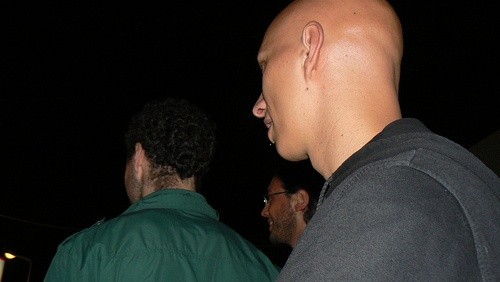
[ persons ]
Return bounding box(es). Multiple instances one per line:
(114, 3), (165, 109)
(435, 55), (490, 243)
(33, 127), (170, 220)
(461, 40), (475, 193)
(259, 159), (327, 252)
(250, 0), (500, 282)
(41, 94), (281, 282)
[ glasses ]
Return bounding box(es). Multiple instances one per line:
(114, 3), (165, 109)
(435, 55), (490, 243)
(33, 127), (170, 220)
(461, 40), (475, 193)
(264, 190), (292, 205)
